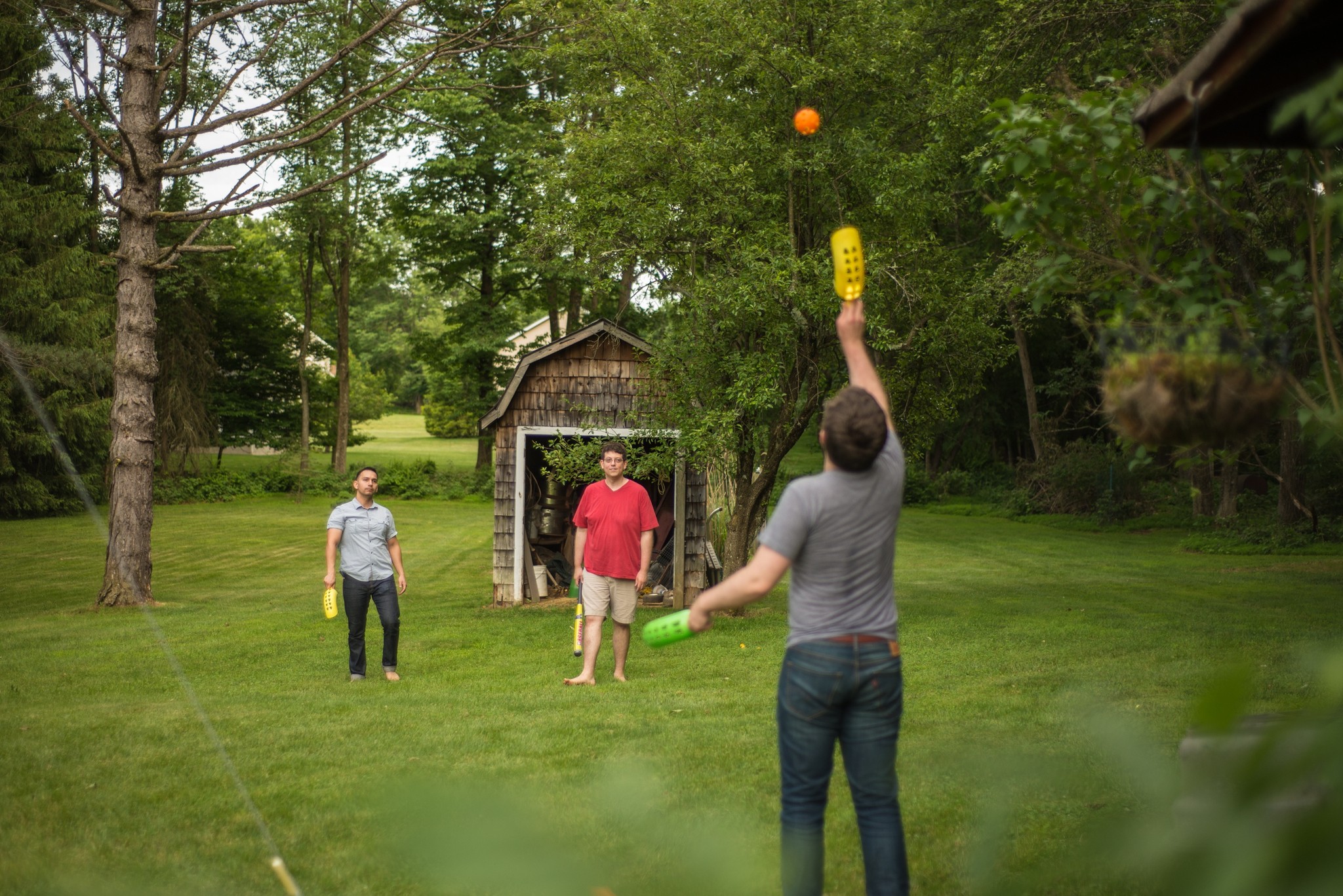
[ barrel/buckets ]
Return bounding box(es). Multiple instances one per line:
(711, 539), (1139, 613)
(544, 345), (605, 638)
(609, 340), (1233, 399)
(567, 574), (579, 598)
(524, 565), (548, 598)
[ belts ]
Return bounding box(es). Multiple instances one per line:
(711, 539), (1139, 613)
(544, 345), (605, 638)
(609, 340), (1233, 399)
(825, 634), (884, 643)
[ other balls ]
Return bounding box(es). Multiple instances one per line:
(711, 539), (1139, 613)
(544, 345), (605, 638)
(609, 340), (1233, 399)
(794, 109), (820, 135)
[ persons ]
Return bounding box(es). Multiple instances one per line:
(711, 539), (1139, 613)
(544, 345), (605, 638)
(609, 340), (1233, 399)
(323, 467), (407, 684)
(562, 449), (660, 686)
(685, 296), (914, 895)
